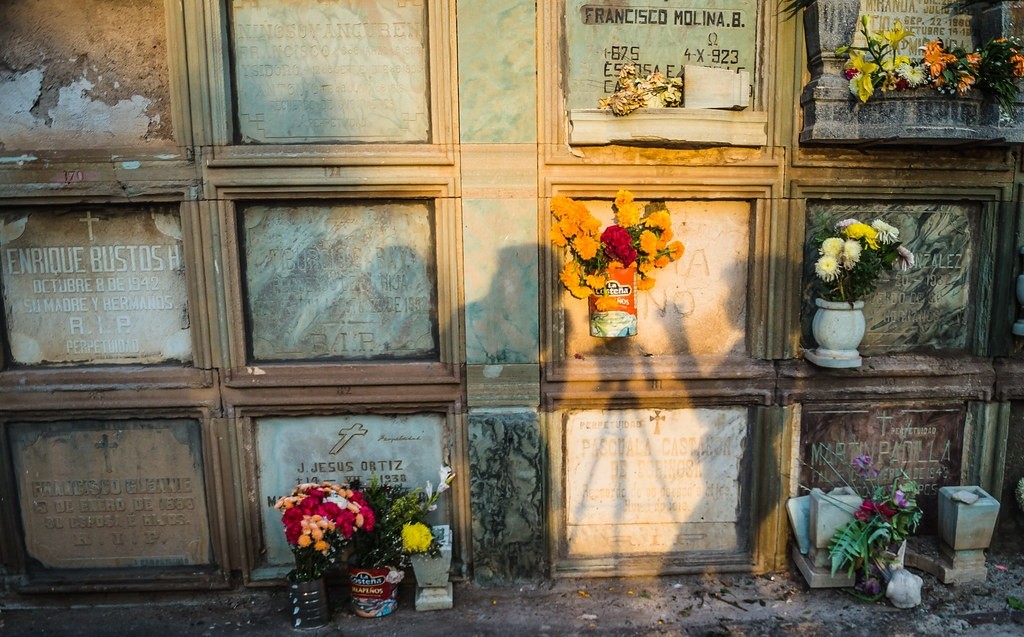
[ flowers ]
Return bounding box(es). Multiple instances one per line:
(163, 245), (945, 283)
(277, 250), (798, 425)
(549, 186), (686, 312)
(598, 61), (683, 117)
(832, 9), (1024, 117)
(792, 436), (923, 603)
(806, 216), (914, 309)
(344, 464), (457, 586)
(273, 479), (377, 583)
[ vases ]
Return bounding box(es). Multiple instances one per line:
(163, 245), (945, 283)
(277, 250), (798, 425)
(586, 267), (638, 338)
(343, 566), (399, 619)
(681, 63), (750, 109)
(886, 543), (925, 609)
(286, 568), (331, 631)
(811, 297), (866, 360)
